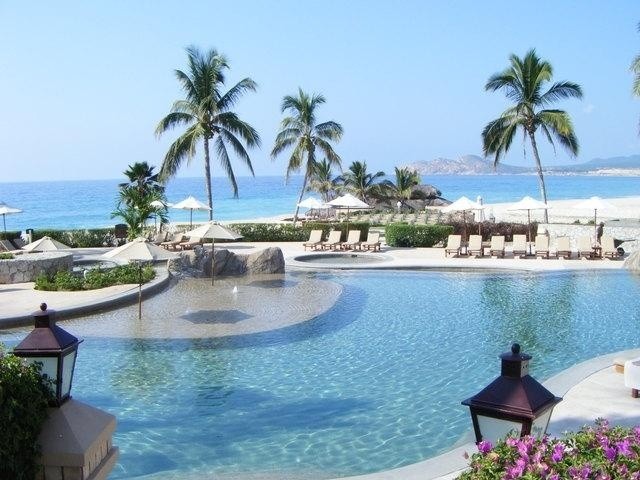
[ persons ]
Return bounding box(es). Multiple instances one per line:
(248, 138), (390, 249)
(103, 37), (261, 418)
(597, 222), (605, 245)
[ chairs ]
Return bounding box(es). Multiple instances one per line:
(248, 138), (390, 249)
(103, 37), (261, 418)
(302, 229), (381, 252)
(444, 234), (619, 261)
(151, 233), (203, 251)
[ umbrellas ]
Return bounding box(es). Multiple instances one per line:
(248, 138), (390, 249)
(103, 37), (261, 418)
(0, 204), (23, 231)
(574, 196), (617, 255)
(22, 236), (71, 253)
(506, 196), (551, 255)
(171, 195), (212, 232)
(443, 196), (485, 255)
(296, 192), (371, 241)
(180, 220), (245, 286)
(101, 236), (182, 319)
(134, 199), (174, 231)
